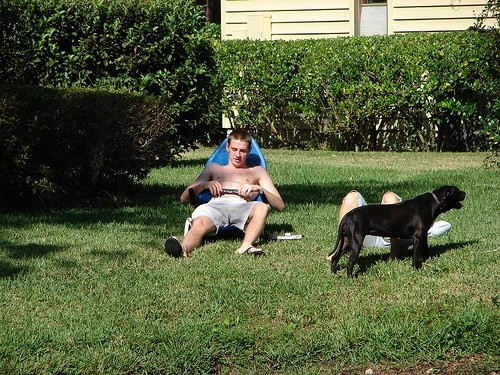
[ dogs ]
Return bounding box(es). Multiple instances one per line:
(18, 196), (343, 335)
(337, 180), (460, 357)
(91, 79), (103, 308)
(327, 185), (466, 280)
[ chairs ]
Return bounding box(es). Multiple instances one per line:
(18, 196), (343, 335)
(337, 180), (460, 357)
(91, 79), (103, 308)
(197, 133), (267, 230)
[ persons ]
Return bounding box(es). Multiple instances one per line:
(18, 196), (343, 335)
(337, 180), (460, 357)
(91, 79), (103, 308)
(163, 127), (284, 258)
(337, 189), (453, 249)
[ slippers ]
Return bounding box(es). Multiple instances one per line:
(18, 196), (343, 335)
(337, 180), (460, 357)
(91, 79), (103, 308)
(165, 236), (187, 260)
(234, 246), (264, 255)
